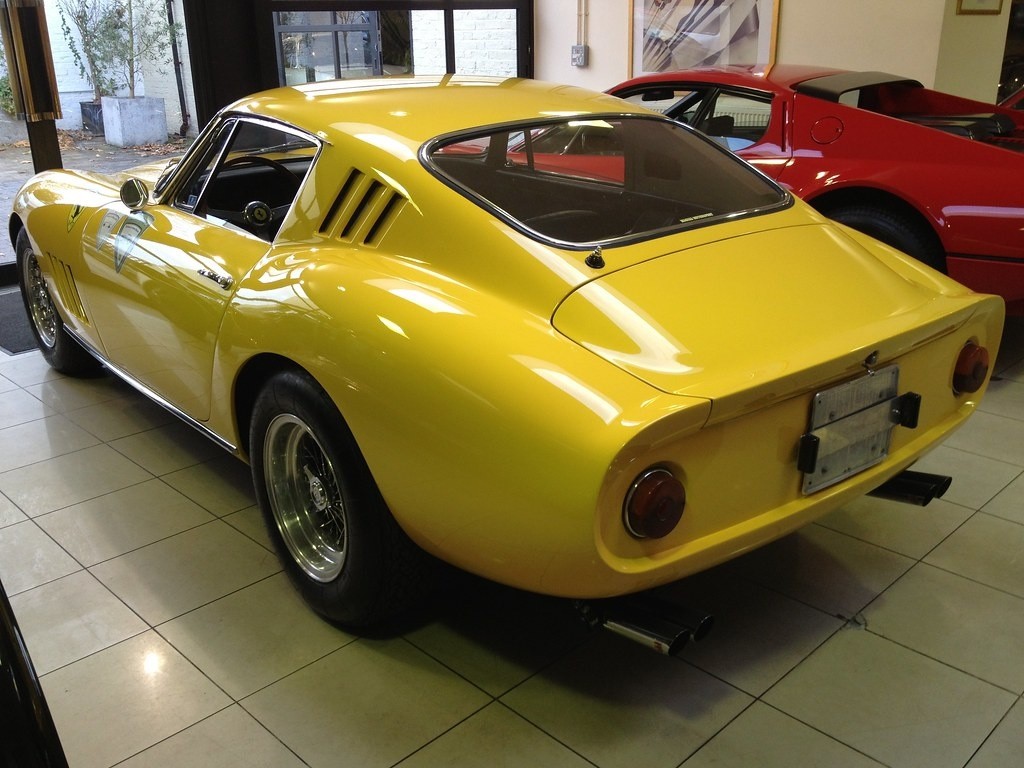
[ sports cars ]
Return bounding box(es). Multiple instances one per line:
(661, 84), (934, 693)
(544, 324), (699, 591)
(7, 70), (1006, 656)
(433, 63), (1024, 311)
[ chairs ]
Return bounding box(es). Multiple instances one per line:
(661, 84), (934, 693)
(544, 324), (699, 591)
(521, 208), (607, 245)
(700, 115), (736, 139)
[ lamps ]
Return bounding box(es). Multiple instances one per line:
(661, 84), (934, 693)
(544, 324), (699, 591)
(570, 45), (588, 68)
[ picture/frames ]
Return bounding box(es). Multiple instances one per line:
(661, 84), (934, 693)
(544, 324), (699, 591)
(956, 0), (1003, 16)
(627, 0), (781, 82)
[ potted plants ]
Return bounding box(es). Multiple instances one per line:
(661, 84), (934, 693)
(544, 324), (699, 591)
(60, 0), (136, 137)
(100, 0), (181, 149)
